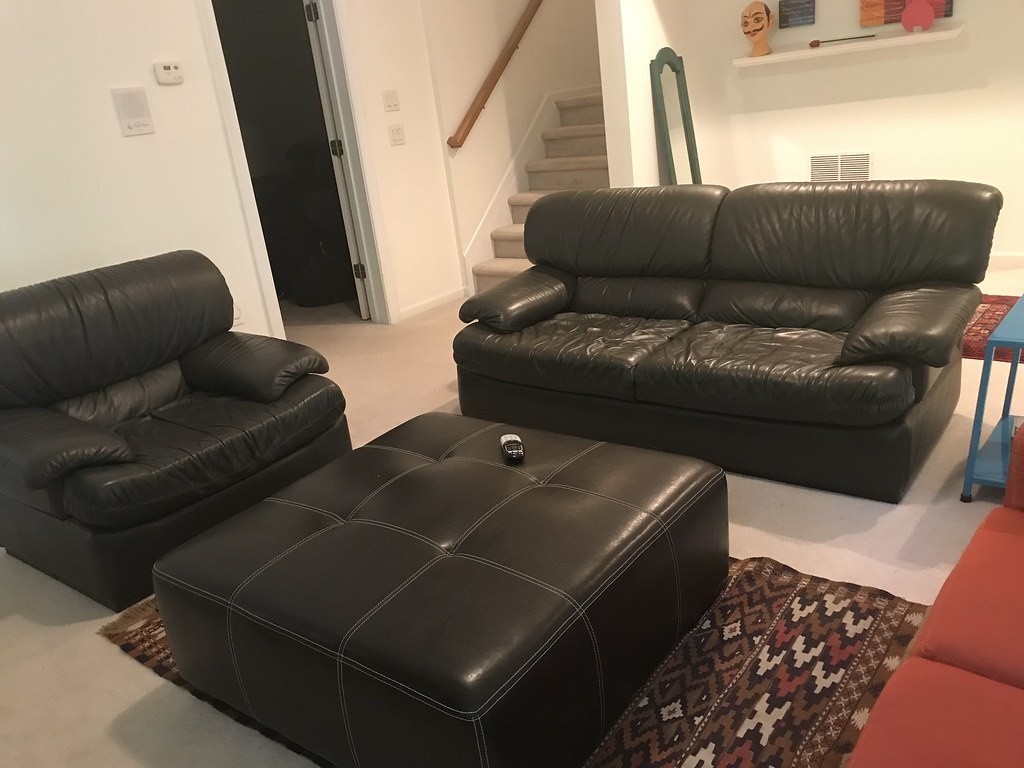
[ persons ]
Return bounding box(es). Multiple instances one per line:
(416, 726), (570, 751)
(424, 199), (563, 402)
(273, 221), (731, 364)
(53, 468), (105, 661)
(741, 1), (775, 57)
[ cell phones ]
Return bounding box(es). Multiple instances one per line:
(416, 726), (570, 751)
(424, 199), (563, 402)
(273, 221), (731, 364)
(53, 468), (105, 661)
(500, 434), (525, 460)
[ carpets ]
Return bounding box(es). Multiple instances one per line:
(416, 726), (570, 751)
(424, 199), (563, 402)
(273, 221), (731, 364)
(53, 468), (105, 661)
(94, 556), (931, 768)
(963, 294), (1024, 364)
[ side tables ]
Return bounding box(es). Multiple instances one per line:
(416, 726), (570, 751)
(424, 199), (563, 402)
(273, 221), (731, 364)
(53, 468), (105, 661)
(960, 294), (1024, 500)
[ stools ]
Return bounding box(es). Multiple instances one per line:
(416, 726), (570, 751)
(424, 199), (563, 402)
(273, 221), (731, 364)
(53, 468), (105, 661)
(151, 411), (729, 768)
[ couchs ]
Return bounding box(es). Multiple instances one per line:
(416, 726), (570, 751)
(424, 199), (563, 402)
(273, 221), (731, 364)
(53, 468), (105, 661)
(452, 179), (1004, 506)
(850, 423), (1024, 768)
(0, 249), (354, 613)
